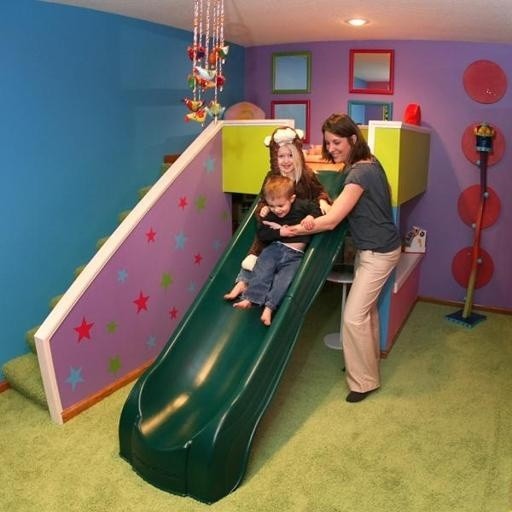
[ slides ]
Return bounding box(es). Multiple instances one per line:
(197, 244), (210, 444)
(118, 166), (350, 505)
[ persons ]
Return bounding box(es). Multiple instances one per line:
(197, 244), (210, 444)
(222, 124), (336, 302)
(230, 174), (317, 328)
(261, 111), (403, 404)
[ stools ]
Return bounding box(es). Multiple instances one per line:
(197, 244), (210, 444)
(324, 264), (354, 350)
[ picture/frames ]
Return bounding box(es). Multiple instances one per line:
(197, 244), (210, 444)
(269, 47), (395, 143)
